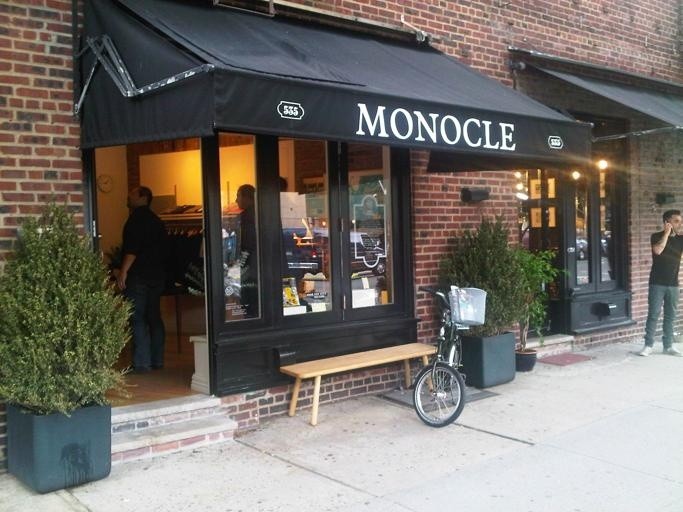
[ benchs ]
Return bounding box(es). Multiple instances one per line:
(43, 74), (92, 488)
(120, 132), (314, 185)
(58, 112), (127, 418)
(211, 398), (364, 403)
(276, 338), (439, 426)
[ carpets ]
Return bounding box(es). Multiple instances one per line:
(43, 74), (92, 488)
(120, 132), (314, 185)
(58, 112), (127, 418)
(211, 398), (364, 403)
(535, 350), (597, 368)
(376, 373), (501, 415)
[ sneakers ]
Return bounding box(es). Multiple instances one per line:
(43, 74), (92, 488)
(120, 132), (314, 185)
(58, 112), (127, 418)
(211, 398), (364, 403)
(126, 363), (164, 374)
(662, 346), (681, 355)
(639, 346), (653, 356)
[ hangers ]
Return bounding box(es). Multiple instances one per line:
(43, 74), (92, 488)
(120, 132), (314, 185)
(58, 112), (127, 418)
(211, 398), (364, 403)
(160, 218), (241, 236)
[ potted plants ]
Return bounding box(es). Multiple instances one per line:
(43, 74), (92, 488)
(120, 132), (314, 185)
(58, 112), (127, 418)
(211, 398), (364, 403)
(512, 243), (573, 374)
(0, 187), (138, 497)
(433, 208), (524, 392)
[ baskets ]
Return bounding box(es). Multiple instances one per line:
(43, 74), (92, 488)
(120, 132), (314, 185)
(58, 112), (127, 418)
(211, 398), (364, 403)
(448, 287), (488, 327)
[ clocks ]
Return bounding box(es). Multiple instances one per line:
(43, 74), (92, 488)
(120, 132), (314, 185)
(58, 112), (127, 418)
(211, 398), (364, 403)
(96, 173), (115, 194)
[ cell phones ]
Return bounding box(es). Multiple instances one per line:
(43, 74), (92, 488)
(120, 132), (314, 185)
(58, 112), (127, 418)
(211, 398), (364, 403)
(665, 220), (674, 234)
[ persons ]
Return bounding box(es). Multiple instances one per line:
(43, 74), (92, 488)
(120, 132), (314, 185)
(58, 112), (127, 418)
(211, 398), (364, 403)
(114, 184), (170, 377)
(278, 176), (302, 229)
(234, 184), (259, 320)
(639, 207), (683, 357)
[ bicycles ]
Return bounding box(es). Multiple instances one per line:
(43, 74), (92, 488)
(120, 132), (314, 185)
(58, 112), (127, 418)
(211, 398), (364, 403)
(411, 284), (486, 426)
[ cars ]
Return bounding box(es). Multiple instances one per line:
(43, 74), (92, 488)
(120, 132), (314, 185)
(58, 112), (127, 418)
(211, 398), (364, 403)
(280, 226), (384, 279)
(573, 225), (611, 262)
